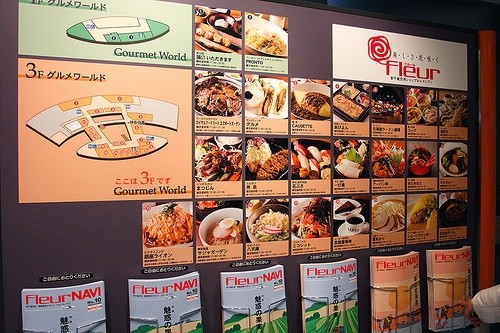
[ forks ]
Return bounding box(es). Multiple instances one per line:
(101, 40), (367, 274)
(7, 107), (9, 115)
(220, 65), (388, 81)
(342, 202), (366, 217)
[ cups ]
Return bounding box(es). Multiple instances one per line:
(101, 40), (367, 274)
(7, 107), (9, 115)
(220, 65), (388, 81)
(345, 213), (370, 234)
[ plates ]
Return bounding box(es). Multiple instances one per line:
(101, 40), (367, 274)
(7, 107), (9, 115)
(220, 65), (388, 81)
(449, 191), (467, 202)
(333, 198), (363, 221)
(338, 222), (370, 237)
(142, 200), (193, 249)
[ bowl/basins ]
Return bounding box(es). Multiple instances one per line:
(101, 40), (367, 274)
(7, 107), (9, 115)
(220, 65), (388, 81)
(439, 198), (467, 228)
(198, 207), (244, 246)
(191, 4), (470, 181)
(292, 198), (331, 240)
(371, 194), (406, 235)
(244, 203), (290, 243)
(195, 201), (238, 220)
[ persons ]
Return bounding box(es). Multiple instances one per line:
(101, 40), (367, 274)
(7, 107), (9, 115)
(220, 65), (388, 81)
(459, 284), (500, 326)
